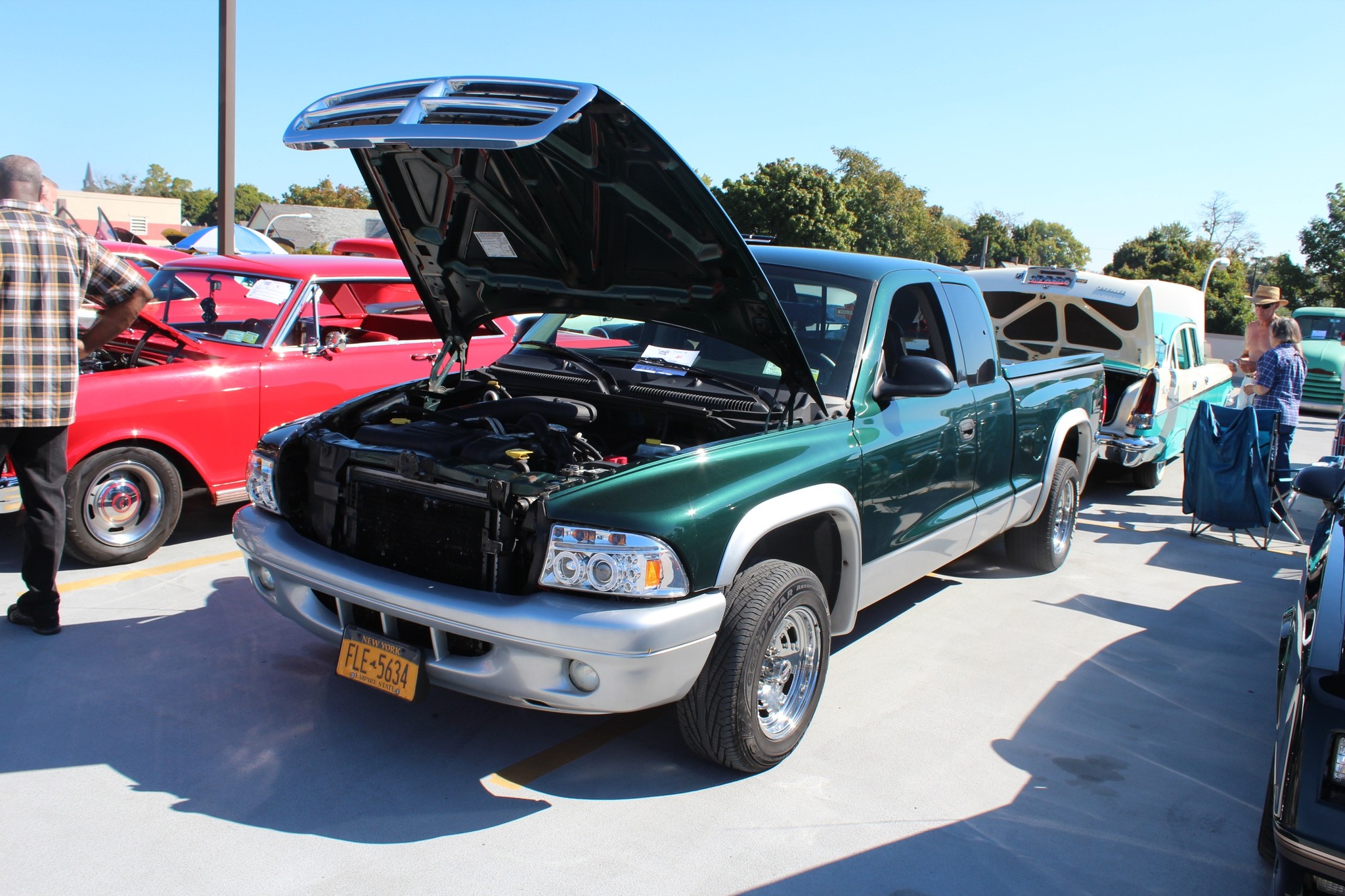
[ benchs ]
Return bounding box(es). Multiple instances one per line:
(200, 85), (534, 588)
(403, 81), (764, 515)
(360, 314), (494, 340)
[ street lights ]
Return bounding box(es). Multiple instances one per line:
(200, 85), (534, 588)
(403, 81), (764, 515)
(264, 204), (312, 236)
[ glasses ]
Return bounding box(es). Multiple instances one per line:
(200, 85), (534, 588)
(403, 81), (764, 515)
(1254, 303), (1271, 309)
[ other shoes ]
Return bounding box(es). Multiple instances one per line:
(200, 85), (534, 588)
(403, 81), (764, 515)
(7, 603), (62, 635)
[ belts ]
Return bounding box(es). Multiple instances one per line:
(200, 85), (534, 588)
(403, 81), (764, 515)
(1246, 375), (1255, 379)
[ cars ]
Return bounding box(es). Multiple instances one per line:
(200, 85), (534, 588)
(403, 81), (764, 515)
(1290, 305), (1345, 408)
(1254, 407), (1345, 896)
(962, 267), (1235, 484)
(0, 237), (634, 564)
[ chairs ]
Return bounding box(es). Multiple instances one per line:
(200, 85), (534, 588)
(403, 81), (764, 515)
(255, 318), (319, 348)
(1181, 398), (1315, 552)
(320, 325), (397, 345)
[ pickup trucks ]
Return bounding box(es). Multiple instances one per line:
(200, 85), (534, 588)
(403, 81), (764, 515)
(226, 75), (1109, 775)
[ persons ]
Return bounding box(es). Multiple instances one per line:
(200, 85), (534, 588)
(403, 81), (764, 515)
(1228, 284), (1307, 524)
(1, 155), (155, 638)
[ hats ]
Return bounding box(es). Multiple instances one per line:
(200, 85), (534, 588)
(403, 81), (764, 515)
(1244, 285), (1288, 306)
